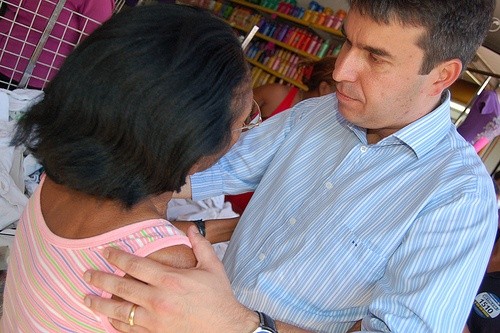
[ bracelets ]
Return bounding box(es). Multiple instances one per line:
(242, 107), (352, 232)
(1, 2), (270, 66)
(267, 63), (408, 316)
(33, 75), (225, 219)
(251, 309), (278, 333)
(192, 218), (205, 237)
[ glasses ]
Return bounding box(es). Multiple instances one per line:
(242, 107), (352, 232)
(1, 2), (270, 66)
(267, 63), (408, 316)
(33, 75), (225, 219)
(228, 98), (262, 133)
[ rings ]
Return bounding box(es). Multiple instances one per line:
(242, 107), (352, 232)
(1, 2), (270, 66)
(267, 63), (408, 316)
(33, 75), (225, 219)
(129, 304), (138, 326)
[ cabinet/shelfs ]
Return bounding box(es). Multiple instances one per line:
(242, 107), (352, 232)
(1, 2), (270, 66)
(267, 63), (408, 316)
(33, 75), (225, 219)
(179, 0), (343, 90)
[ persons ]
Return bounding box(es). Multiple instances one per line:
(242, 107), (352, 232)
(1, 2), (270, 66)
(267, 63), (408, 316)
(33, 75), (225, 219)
(173, 0), (499, 333)
(0, 2), (253, 333)
(0, 0), (114, 91)
(223, 55), (342, 213)
